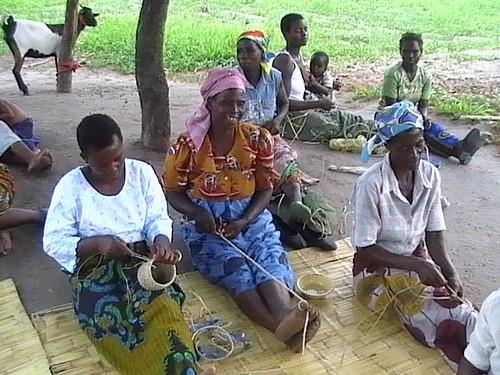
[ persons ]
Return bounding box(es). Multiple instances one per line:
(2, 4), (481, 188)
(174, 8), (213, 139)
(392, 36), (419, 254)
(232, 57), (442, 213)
(350, 101), (480, 373)
(42, 114), (215, 375)
(0, 99), (53, 257)
(457, 287), (500, 375)
(162, 68), (321, 353)
(232, 13), (486, 225)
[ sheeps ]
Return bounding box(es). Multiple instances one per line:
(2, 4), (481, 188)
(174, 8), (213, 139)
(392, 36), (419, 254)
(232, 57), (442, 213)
(1, 2), (100, 96)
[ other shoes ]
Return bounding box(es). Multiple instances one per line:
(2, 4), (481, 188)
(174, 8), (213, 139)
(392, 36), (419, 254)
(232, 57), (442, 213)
(271, 213), (308, 249)
(462, 133), (486, 165)
(299, 222), (338, 250)
(458, 128), (481, 162)
(288, 201), (311, 227)
